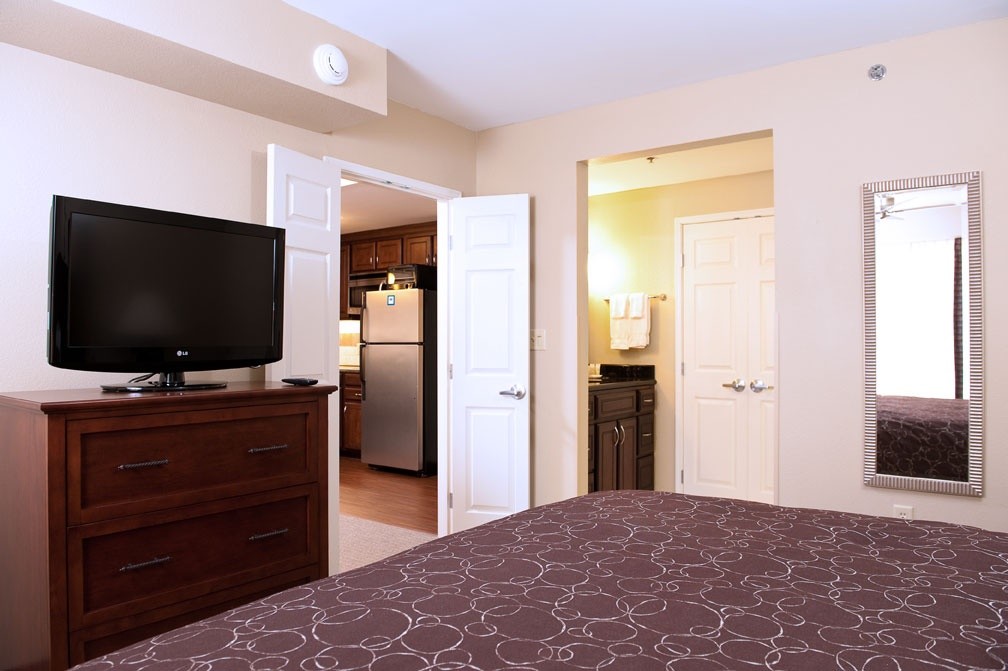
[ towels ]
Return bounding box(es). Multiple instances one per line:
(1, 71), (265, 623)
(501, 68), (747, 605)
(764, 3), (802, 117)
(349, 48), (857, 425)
(609, 290), (651, 350)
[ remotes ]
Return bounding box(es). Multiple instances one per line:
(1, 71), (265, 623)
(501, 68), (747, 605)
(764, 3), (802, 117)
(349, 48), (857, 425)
(282, 378), (319, 386)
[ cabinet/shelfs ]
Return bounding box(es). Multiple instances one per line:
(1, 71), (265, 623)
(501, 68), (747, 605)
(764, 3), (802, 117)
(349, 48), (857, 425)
(588, 380), (657, 497)
(338, 370), (362, 457)
(0, 381), (338, 671)
(340, 220), (437, 320)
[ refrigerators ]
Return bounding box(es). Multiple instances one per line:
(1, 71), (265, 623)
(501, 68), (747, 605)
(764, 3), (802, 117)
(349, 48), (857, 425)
(359, 287), (436, 476)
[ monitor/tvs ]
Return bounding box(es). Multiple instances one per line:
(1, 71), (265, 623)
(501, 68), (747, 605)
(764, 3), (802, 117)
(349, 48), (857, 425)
(46, 195), (286, 391)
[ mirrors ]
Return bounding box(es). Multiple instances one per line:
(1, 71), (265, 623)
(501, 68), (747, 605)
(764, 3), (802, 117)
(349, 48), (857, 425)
(863, 171), (982, 498)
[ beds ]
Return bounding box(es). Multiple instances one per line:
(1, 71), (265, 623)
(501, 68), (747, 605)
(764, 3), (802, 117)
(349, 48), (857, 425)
(57, 487), (1008, 671)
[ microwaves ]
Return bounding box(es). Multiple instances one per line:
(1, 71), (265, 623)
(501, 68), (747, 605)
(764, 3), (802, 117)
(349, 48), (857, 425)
(347, 267), (438, 314)
